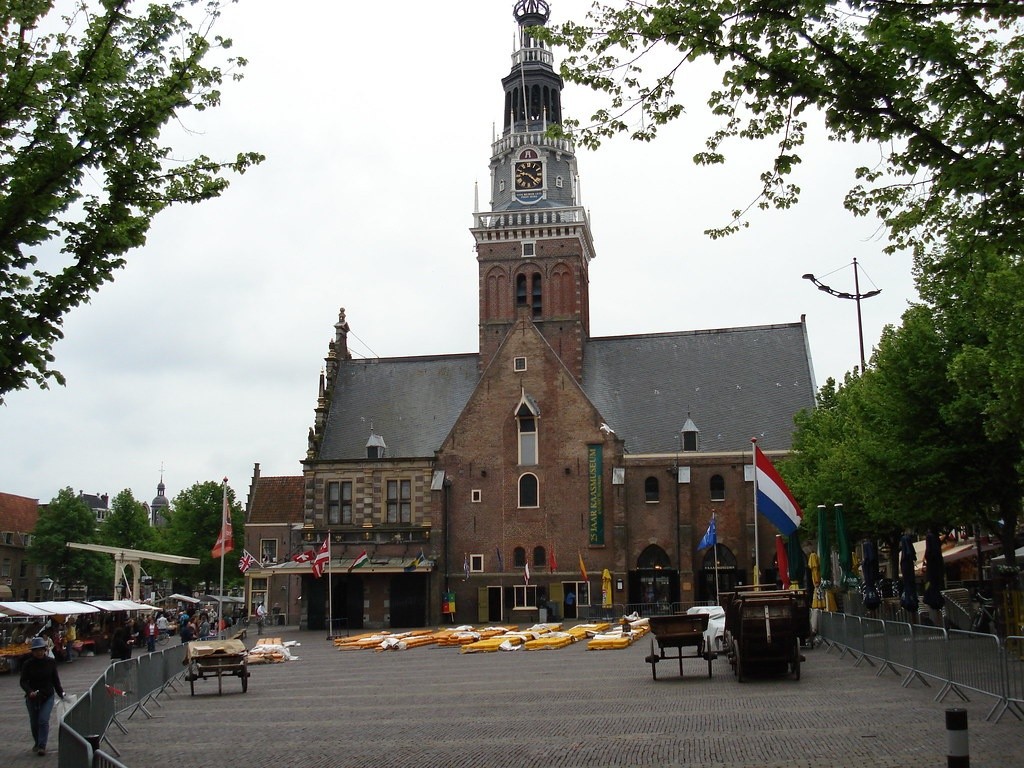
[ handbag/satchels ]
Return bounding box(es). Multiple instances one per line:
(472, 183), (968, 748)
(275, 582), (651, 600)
(57, 693), (77, 727)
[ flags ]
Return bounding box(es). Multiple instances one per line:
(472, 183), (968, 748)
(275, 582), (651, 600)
(238, 551), (255, 572)
(464, 559), (469, 578)
(404, 551), (424, 572)
(312, 538), (328, 578)
(550, 550), (557, 574)
(525, 563), (530, 581)
(348, 550), (368, 573)
(697, 518), (717, 550)
(756, 446), (802, 536)
(293, 550), (314, 563)
(212, 499), (234, 557)
(579, 555), (588, 581)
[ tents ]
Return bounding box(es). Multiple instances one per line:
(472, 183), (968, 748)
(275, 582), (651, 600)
(0, 600), (163, 615)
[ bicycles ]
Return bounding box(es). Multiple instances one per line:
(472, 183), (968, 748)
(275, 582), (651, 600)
(154, 630), (171, 646)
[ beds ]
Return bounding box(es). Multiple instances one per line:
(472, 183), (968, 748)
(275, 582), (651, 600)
(257, 637), (283, 662)
(335, 616), (650, 651)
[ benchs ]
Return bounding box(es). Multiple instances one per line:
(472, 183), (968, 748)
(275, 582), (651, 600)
(233, 629), (247, 638)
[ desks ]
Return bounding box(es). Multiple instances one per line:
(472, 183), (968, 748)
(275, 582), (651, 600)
(80, 639), (95, 656)
(0, 650), (31, 676)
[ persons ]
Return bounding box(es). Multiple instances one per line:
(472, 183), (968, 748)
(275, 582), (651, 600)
(14, 617), (76, 663)
(20, 637), (64, 755)
(179, 608), (231, 643)
(258, 601), (267, 634)
(111, 614), (169, 663)
(566, 592), (576, 618)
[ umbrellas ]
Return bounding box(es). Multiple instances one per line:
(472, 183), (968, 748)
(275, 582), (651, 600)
(776, 504), (947, 613)
(602, 568), (613, 608)
(753, 566), (757, 591)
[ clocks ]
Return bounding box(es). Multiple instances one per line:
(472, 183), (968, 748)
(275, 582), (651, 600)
(514, 162), (543, 188)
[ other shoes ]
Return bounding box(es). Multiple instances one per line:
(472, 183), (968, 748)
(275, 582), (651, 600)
(32, 744), (38, 751)
(38, 749), (46, 757)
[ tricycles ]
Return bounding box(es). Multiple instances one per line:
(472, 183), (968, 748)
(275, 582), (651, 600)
(182, 634), (250, 696)
(643, 610), (718, 681)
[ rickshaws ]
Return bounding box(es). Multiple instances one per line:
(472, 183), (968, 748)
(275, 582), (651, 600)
(718, 581), (815, 684)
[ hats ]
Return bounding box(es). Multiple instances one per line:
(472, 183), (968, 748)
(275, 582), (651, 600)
(28, 637), (46, 649)
